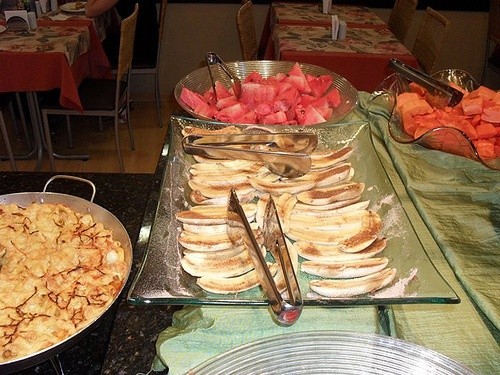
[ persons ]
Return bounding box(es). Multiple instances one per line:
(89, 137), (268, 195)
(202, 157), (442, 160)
(85, 0), (158, 124)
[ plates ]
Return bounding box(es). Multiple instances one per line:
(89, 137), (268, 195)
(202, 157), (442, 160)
(60, 2), (88, 13)
(176, 328), (478, 375)
(367, 68), (500, 173)
(0, 25), (6, 33)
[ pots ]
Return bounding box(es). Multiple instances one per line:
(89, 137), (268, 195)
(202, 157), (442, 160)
(0, 175), (134, 371)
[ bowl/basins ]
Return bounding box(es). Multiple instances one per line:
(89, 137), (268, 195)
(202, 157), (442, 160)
(173, 60), (361, 127)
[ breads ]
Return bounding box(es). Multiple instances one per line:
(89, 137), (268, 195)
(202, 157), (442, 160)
(175, 125), (397, 297)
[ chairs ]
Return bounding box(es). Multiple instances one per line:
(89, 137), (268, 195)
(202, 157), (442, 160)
(387, 0), (417, 41)
(0, 0), (169, 172)
(237, 1), (258, 61)
(412, 7), (449, 75)
(481, 0), (500, 83)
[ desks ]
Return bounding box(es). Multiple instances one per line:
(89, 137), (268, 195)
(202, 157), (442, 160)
(259, 2), (417, 94)
(0, 0), (113, 161)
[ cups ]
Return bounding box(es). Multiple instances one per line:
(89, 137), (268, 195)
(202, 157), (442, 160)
(27, 12), (37, 29)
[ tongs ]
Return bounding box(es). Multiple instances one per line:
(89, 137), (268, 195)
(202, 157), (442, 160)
(205, 51), (242, 104)
(229, 186), (304, 327)
(181, 128), (318, 179)
(388, 57), (464, 107)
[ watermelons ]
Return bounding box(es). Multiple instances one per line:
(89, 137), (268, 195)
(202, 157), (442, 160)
(396, 82), (500, 158)
(180, 62), (342, 125)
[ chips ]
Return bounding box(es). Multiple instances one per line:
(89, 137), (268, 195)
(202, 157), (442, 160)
(0, 203), (125, 362)
